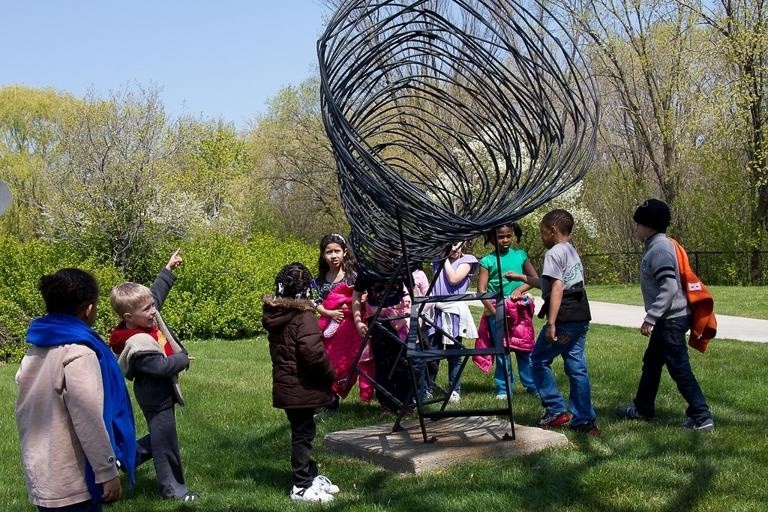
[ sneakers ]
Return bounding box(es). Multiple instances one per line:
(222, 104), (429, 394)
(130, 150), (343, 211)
(534, 410), (570, 429)
(290, 485), (335, 504)
(380, 406), (397, 420)
(526, 388), (541, 400)
(448, 390), (460, 403)
(561, 425), (601, 436)
(496, 394), (513, 401)
(614, 404), (656, 421)
(311, 474), (340, 494)
(678, 416), (715, 432)
(313, 407), (335, 419)
(401, 389), (433, 420)
(178, 493), (206, 501)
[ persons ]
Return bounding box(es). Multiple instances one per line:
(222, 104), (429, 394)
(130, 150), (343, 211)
(261, 262), (340, 504)
(503, 209), (600, 437)
(109, 247), (199, 502)
(615, 199), (717, 433)
(15, 268), (137, 512)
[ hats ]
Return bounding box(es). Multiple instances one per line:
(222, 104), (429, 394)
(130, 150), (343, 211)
(633, 199), (671, 232)
